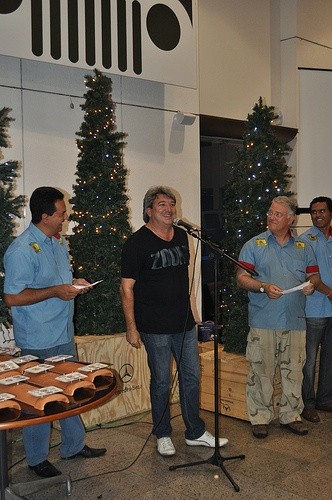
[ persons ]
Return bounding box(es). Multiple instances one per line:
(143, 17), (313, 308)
(3, 186), (108, 479)
(299, 196), (332, 423)
(236, 196), (321, 438)
(120, 186), (230, 456)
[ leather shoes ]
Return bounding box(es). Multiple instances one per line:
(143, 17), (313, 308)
(61, 445), (107, 460)
(28, 460), (62, 478)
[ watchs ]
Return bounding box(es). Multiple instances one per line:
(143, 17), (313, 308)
(260, 281), (265, 292)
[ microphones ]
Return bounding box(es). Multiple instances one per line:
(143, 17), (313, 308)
(173, 217), (197, 230)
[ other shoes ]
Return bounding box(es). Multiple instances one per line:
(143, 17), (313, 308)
(252, 424), (268, 438)
(315, 402), (332, 412)
(279, 421), (308, 435)
(302, 410), (320, 422)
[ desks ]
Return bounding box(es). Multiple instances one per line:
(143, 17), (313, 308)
(0, 354), (123, 500)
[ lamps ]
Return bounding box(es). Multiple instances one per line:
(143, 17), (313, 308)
(175, 112), (196, 127)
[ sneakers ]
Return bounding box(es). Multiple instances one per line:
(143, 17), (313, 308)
(156, 436), (176, 456)
(185, 430), (228, 448)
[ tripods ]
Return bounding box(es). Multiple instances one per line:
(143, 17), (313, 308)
(168, 230), (259, 492)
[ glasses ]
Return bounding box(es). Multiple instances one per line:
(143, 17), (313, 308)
(266, 211), (288, 218)
